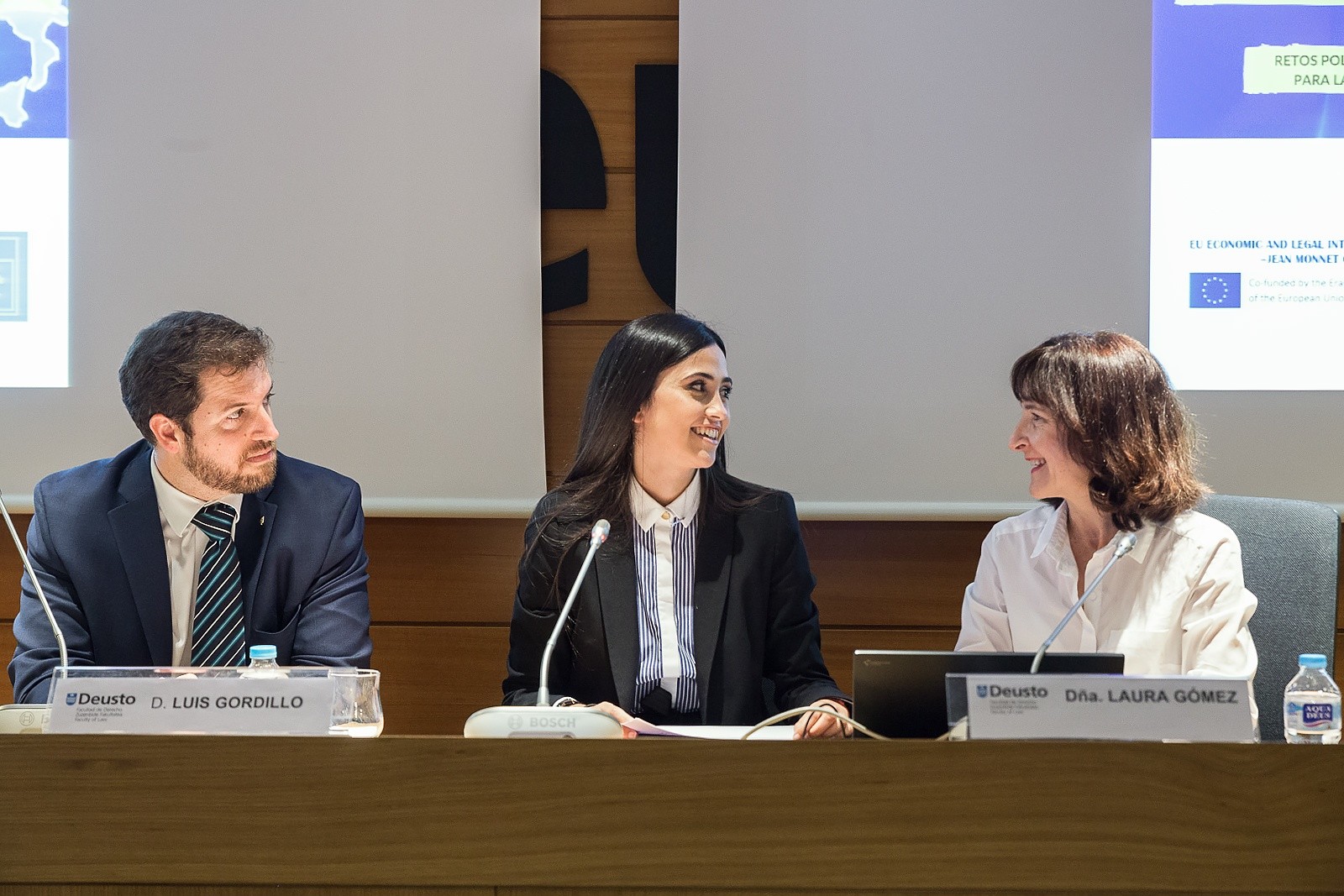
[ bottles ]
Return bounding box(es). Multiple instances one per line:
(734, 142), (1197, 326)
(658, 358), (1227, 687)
(1284, 653), (1342, 744)
(240, 644), (289, 679)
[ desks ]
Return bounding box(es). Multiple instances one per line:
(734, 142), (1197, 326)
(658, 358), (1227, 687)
(0, 731), (1344, 896)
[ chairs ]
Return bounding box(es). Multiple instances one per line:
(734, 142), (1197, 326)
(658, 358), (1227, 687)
(1182, 495), (1341, 741)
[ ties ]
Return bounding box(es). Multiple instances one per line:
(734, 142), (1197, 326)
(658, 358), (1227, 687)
(191, 502), (247, 668)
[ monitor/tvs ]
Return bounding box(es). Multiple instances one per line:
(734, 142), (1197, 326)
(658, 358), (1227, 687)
(851, 650), (1128, 739)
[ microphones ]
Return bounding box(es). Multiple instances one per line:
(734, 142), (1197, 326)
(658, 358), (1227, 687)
(463, 518), (625, 740)
(1032, 533), (1138, 676)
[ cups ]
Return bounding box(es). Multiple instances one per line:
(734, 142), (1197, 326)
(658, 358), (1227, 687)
(326, 668), (385, 738)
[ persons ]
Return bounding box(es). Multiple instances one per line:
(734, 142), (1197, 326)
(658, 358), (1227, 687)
(954, 330), (1259, 742)
(7, 310), (373, 704)
(501, 312), (853, 741)
